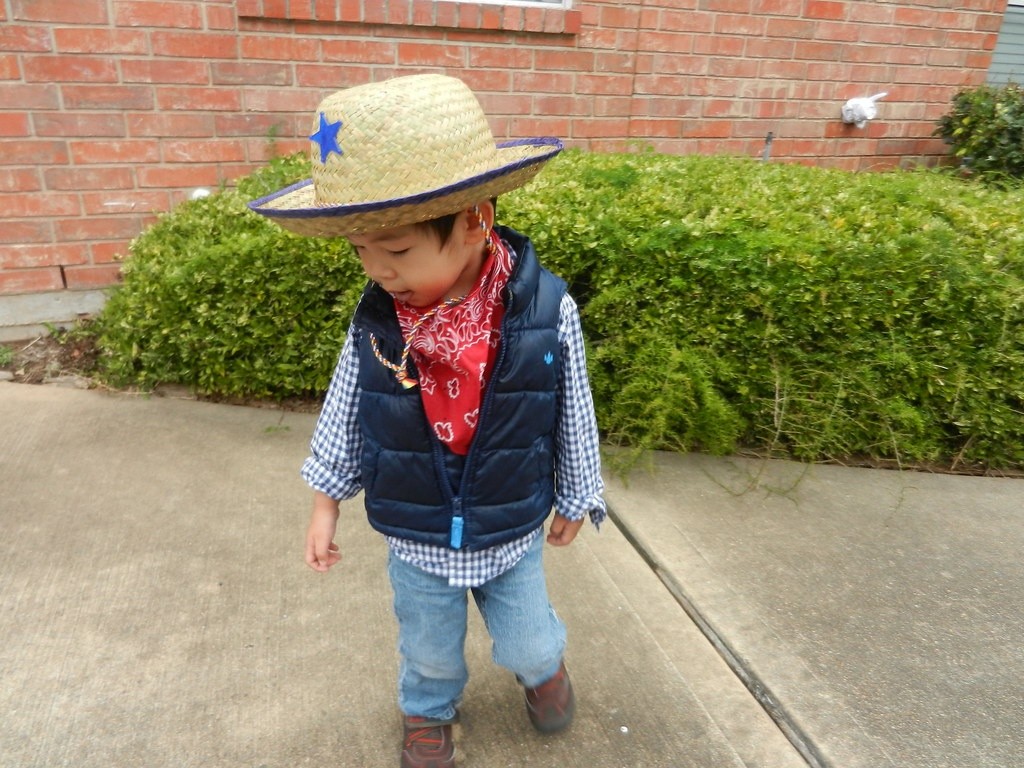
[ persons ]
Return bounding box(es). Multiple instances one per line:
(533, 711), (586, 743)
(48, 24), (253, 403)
(249, 73), (606, 768)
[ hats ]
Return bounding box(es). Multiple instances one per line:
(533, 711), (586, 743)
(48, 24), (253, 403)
(247, 74), (566, 237)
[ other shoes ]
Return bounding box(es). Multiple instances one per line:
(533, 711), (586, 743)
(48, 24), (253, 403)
(522, 660), (576, 734)
(400, 704), (462, 768)
